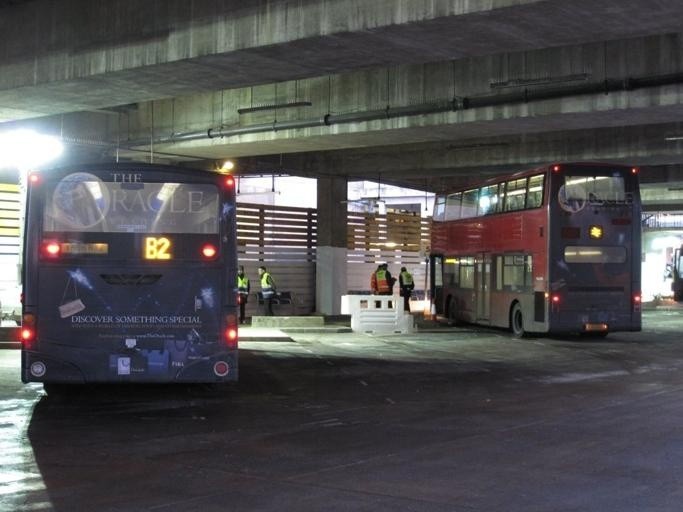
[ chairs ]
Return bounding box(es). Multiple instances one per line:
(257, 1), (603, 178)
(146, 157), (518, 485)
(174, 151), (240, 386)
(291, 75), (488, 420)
(255, 291), (292, 316)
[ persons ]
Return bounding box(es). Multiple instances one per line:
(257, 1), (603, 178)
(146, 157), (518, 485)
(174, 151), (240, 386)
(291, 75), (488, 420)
(370, 264), (379, 295)
(398, 266), (414, 313)
(237, 264), (250, 325)
(375, 263), (397, 308)
(257, 266), (277, 315)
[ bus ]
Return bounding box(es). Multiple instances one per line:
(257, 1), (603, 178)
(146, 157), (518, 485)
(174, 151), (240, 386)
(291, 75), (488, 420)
(429, 161), (641, 340)
(22, 162), (239, 391)
